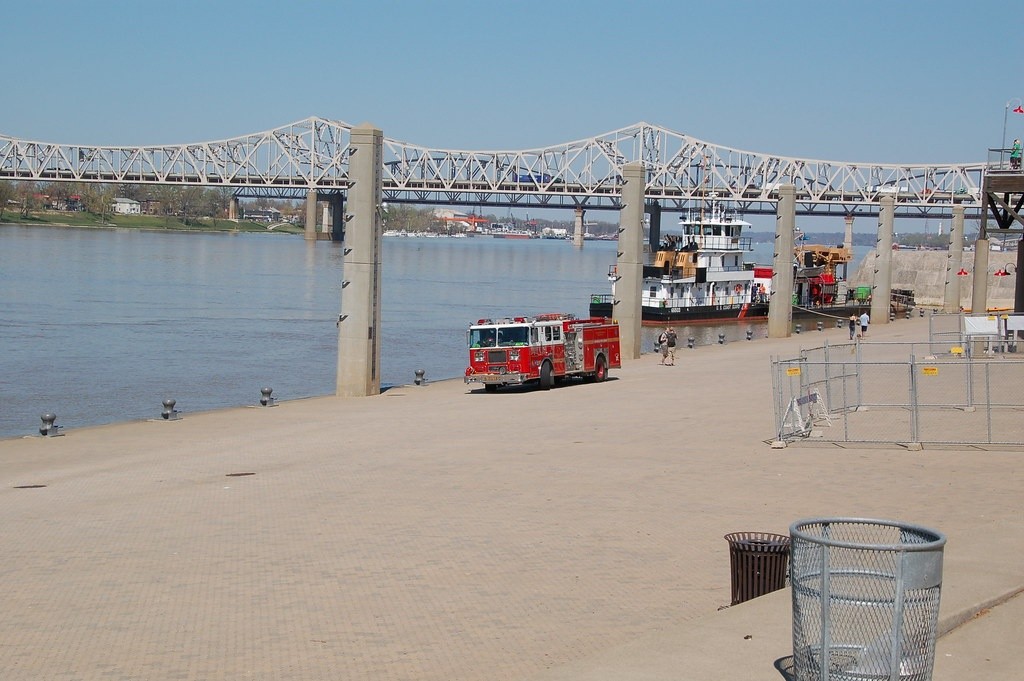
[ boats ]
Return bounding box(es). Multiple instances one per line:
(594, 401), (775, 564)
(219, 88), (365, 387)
(588, 198), (916, 325)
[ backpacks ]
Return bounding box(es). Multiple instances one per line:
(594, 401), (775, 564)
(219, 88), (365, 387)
(667, 333), (675, 347)
(658, 332), (669, 344)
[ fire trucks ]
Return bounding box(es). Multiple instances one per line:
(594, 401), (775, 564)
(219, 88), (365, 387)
(463, 312), (622, 390)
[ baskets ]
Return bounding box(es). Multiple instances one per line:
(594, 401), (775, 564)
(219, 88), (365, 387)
(774, 653), (857, 681)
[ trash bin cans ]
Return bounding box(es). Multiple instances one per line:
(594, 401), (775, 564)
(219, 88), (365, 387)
(787, 516), (949, 681)
(722, 529), (792, 608)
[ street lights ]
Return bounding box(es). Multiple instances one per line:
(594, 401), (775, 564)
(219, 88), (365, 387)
(999, 97), (1024, 174)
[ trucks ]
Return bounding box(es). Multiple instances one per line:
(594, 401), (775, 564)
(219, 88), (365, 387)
(857, 184), (909, 193)
(514, 173), (564, 184)
(921, 188), (957, 194)
(748, 182), (782, 190)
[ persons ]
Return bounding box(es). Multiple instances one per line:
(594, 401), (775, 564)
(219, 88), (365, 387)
(1009, 139), (1020, 169)
(658, 327), (678, 366)
(752, 283), (765, 303)
(478, 333), (502, 347)
(662, 233), (682, 250)
(848, 310), (869, 340)
